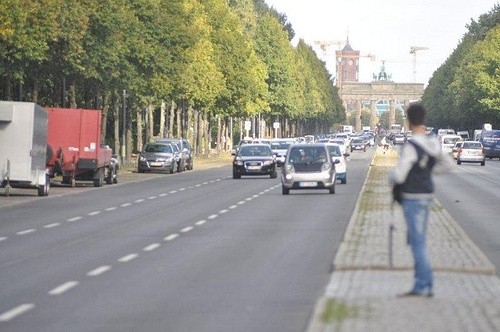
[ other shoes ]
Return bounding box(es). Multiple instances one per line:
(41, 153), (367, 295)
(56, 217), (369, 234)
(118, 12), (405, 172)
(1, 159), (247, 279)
(396, 292), (435, 301)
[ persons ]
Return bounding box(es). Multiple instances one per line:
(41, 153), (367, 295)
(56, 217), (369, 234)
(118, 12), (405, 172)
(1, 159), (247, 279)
(381, 136), (391, 154)
(389, 103), (453, 298)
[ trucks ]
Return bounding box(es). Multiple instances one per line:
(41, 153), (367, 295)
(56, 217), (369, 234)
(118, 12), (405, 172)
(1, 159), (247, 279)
(0, 101), (56, 196)
(44, 108), (121, 187)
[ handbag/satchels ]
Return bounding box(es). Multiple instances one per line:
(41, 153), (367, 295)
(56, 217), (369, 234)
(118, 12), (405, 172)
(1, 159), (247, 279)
(393, 184), (402, 205)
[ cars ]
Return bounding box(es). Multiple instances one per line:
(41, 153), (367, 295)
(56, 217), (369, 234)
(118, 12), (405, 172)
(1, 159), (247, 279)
(231, 144), (279, 179)
(155, 139), (195, 169)
(239, 124), (412, 153)
(479, 130), (500, 160)
(325, 142), (350, 183)
(136, 143), (183, 171)
(458, 141), (486, 167)
(277, 144), (341, 191)
(425, 126), (485, 158)
